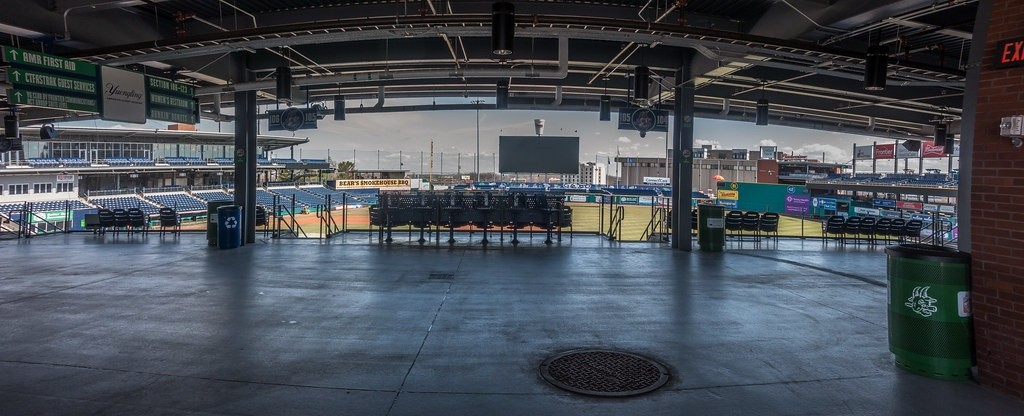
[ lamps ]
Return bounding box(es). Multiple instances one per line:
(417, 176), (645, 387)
(944, 121), (958, 154)
(332, 83), (347, 121)
(863, 23), (892, 93)
(495, 77), (510, 109)
(275, 45), (294, 101)
(933, 106), (948, 147)
(756, 80), (769, 125)
(632, 43), (650, 101)
(490, 0), (516, 56)
(599, 79), (612, 121)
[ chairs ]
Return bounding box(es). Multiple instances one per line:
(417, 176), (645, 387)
(0, 157), (959, 251)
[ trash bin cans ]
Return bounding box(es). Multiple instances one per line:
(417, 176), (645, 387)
(217, 204), (242, 249)
(696, 204), (725, 251)
(883, 242), (977, 380)
(206, 199), (234, 246)
